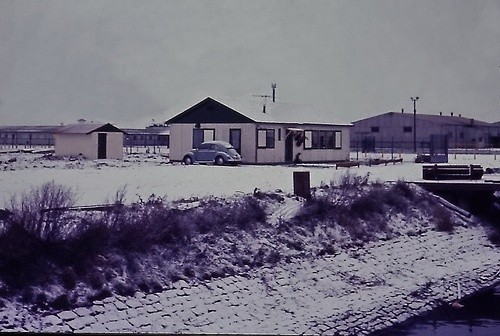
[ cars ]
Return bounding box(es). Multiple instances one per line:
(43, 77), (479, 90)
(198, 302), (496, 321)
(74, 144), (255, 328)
(181, 140), (242, 166)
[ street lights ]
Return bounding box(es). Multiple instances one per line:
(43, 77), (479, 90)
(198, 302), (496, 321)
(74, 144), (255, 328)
(411, 96), (419, 153)
